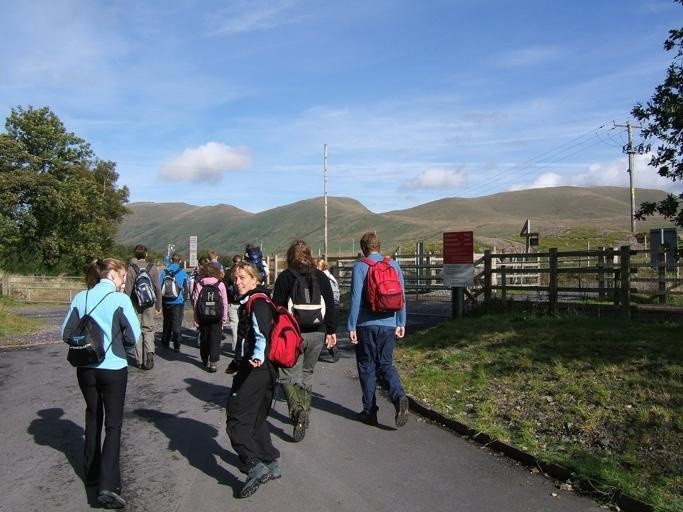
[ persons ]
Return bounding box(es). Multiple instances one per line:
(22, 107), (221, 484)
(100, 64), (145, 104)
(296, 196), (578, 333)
(59, 256), (143, 511)
(269, 239), (338, 442)
(159, 254), (190, 353)
(122, 243), (163, 372)
(220, 260), (281, 499)
(315, 256), (341, 361)
(189, 242), (281, 373)
(346, 231), (409, 429)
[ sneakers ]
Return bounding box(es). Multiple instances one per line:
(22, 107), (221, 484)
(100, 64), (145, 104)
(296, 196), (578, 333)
(137, 365), (144, 368)
(146, 353), (153, 370)
(293, 405), (307, 442)
(394, 396), (409, 427)
(304, 406), (311, 428)
(266, 460), (281, 479)
(356, 411), (377, 426)
(240, 463), (271, 498)
(174, 348), (180, 353)
(97, 490), (126, 509)
(329, 345), (339, 362)
(163, 345), (169, 349)
(85, 477), (99, 486)
(202, 362), (209, 368)
(210, 363), (217, 372)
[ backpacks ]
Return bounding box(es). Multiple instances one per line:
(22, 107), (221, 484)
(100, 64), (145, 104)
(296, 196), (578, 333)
(195, 279), (223, 322)
(130, 263), (156, 307)
(161, 268), (183, 300)
(359, 256), (403, 313)
(247, 293), (304, 368)
(67, 290), (121, 368)
(287, 267), (326, 326)
